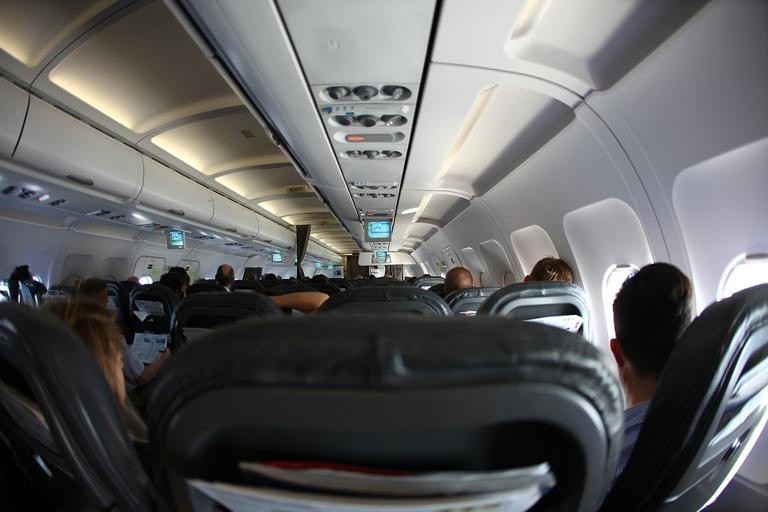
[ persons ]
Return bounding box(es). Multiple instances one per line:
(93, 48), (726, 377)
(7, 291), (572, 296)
(128, 257), (391, 319)
(78, 277), (171, 392)
(524, 257), (575, 287)
(10, 265), (33, 282)
(607, 262), (694, 496)
(37, 299), (154, 474)
(445, 266), (474, 298)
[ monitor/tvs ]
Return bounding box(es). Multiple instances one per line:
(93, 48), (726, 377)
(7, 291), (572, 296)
(364, 217), (391, 242)
(166, 229), (185, 250)
(272, 251), (334, 271)
(376, 251), (387, 263)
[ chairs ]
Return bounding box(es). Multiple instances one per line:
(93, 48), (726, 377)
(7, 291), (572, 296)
(0, 275), (768, 511)
